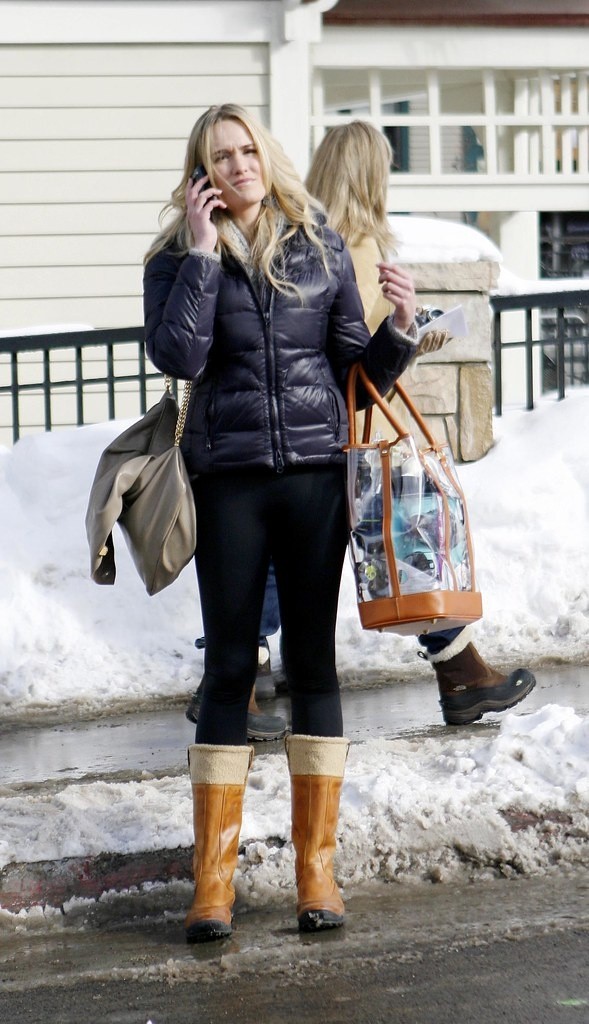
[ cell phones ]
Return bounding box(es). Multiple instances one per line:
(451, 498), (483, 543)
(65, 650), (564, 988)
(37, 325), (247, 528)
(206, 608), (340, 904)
(191, 165), (214, 206)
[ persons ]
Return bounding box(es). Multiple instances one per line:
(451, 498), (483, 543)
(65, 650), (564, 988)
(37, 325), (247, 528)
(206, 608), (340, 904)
(144, 104), (419, 937)
(186, 124), (538, 730)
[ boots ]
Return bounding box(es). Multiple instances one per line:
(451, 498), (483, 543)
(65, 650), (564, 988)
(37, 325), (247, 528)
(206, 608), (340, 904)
(283, 734), (349, 930)
(183, 744), (255, 941)
(417, 625), (537, 725)
(186, 637), (286, 741)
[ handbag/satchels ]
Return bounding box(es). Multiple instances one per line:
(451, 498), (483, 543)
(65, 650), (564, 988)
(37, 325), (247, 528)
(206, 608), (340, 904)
(85, 393), (196, 596)
(339, 365), (484, 636)
